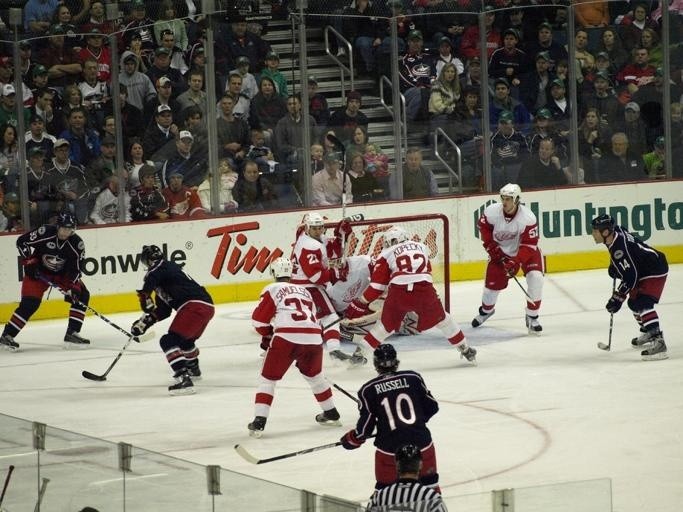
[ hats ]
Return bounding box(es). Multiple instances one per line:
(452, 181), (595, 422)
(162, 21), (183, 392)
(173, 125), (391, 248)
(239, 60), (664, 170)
(5, 84), (15, 96)
(324, 152), (340, 162)
(19, 40), (32, 48)
(154, 104), (171, 115)
(155, 47), (169, 55)
(308, 75), (318, 86)
(124, 54), (137, 61)
(138, 165), (161, 180)
(155, 77), (172, 87)
(347, 92), (361, 101)
(33, 65), (48, 74)
(176, 130), (194, 142)
(53, 139), (70, 150)
(87, 29), (101, 38)
(193, 48), (203, 55)
(101, 135), (115, 145)
(49, 25), (64, 34)
(237, 56), (250, 66)
(407, 6), (641, 124)
(29, 147), (44, 156)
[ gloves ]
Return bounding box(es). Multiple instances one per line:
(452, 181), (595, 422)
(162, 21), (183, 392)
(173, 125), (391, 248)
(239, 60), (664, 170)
(70, 282), (81, 305)
(24, 258), (40, 279)
(131, 313), (154, 335)
(342, 430), (366, 449)
(136, 289), (158, 312)
(260, 335), (272, 350)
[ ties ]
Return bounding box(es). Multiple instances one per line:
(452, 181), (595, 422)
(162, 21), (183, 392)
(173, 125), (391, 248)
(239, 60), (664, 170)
(42, 111), (46, 129)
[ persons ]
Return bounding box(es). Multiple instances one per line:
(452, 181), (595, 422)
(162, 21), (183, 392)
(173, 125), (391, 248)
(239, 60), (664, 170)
(129, 244), (216, 390)
(259, 212), (477, 362)
(1, 0), (439, 234)
(340, 342), (441, 494)
(470, 183), (546, 332)
(365, 443), (449, 512)
(590, 212), (670, 356)
(247, 256), (340, 431)
(0, 213), (90, 348)
(427, 1), (683, 195)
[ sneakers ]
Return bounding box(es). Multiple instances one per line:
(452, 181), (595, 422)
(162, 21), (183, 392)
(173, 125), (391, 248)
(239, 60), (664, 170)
(329, 350), (351, 359)
(472, 306), (495, 327)
(169, 368), (194, 390)
(632, 328), (652, 345)
(0, 332), (19, 346)
(641, 332), (667, 355)
(248, 416), (266, 431)
(526, 314), (542, 331)
(462, 347), (476, 361)
(349, 347), (367, 365)
(316, 407), (340, 422)
(64, 330), (90, 344)
(184, 358), (200, 376)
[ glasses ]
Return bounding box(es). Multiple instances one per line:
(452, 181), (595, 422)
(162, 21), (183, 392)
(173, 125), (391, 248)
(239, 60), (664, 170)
(163, 31), (173, 35)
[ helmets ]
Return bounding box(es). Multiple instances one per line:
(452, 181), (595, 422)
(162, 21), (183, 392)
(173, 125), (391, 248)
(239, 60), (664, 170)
(500, 183), (521, 202)
(398, 443), (422, 462)
(373, 344), (397, 367)
(592, 214), (616, 229)
(57, 213), (79, 227)
(303, 212), (325, 236)
(270, 257), (293, 278)
(383, 232), (404, 248)
(142, 245), (163, 259)
